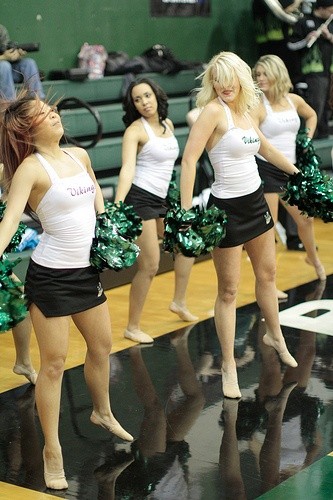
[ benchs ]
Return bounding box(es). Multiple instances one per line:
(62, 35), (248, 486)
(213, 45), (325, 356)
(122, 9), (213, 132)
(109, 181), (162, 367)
(0, 65), (333, 223)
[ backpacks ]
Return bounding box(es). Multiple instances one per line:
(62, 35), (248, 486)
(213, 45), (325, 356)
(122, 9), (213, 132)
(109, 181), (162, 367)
(67, 42), (179, 81)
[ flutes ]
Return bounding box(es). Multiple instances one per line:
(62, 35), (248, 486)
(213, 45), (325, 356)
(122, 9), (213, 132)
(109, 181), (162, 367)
(306, 12), (333, 49)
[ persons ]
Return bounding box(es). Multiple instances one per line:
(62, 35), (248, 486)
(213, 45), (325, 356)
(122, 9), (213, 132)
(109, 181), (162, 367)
(0, 84), (133, 490)
(0, 23), (46, 104)
(172, 51), (327, 399)
(0, 279), (333, 500)
(251, 0), (332, 139)
(111, 78), (199, 343)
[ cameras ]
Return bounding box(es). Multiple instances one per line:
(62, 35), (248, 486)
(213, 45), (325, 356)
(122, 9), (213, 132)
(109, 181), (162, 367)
(7, 42), (39, 52)
